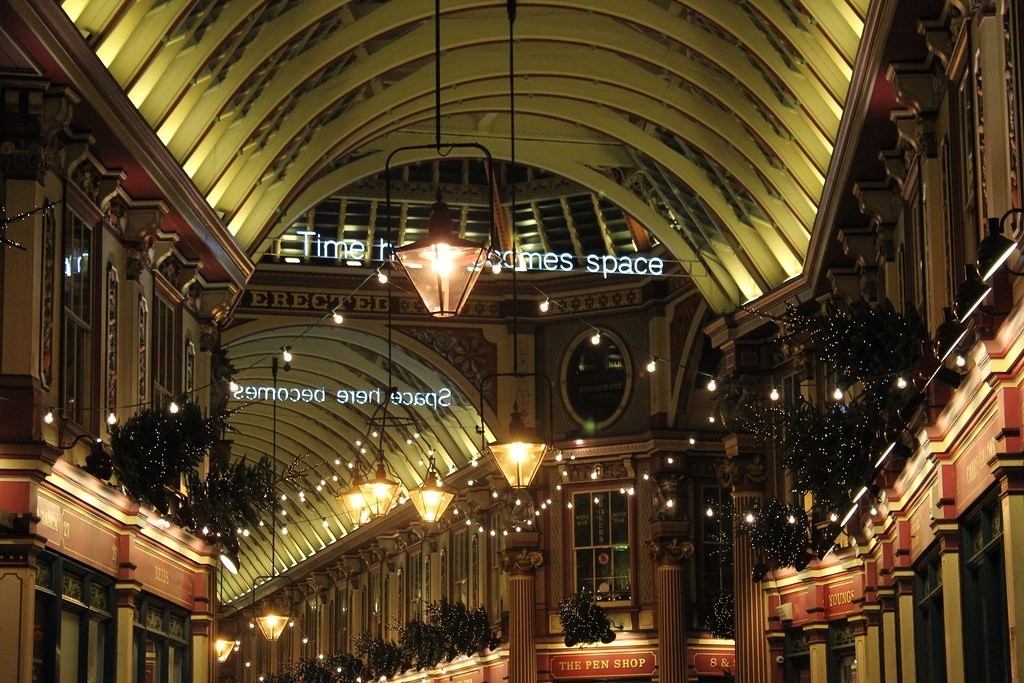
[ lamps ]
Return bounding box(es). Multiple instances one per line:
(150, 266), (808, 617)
(934, 305), (974, 363)
(219, 550), (240, 575)
(54, 433), (113, 481)
(951, 265), (1012, 323)
(140, 492), (195, 528)
(478, 1), (556, 490)
(331, 243), (455, 524)
(976, 208), (1024, 281)
(215, 604), (239, 662)
(251, 352), (294, 642)
(752, 341), (963, 583)
(392, 2), (489, 318)
